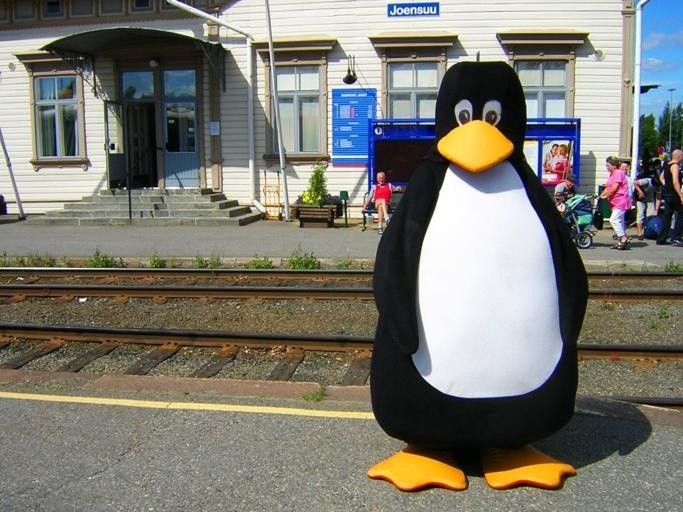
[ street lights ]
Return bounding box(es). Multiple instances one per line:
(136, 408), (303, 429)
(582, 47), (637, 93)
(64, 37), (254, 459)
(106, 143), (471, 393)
(665, 86), (676, 152)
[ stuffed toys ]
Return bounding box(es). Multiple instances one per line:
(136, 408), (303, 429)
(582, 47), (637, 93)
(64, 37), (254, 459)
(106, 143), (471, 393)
(365, 62), (588, 491)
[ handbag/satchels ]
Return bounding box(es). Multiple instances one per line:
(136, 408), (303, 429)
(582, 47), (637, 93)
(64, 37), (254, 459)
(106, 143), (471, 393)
(593, 211), (604, 230)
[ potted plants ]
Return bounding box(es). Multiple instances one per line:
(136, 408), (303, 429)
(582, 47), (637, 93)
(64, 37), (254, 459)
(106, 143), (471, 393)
(294, 160), (343, 230)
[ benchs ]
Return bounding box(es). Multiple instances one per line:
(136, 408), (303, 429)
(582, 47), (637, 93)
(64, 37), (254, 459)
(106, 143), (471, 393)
(359, 190), (405, 232)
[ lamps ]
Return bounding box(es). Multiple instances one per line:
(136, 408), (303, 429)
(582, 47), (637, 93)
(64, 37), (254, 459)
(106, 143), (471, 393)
(342, 55), (356, 84)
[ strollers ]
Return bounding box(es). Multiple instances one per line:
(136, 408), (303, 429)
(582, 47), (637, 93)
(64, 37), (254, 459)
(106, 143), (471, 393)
(556, 191), (600, 249)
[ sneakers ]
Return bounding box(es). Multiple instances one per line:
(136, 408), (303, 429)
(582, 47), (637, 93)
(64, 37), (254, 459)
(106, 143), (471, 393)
(638, 234), (683, 245)
(611, 235), (632, 250)
(377, 220), (391, 234)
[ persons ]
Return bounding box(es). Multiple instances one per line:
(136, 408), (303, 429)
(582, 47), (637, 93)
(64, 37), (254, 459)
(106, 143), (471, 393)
(544, 144), (570, 182)
(364, 172), (402, 234)
(554, 146), (683, 249)
